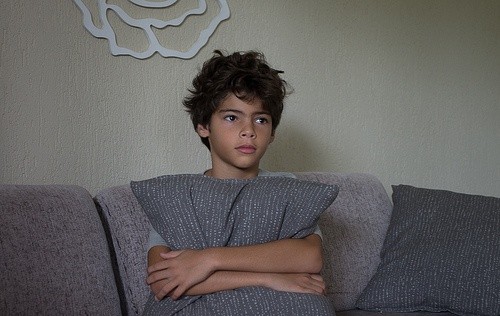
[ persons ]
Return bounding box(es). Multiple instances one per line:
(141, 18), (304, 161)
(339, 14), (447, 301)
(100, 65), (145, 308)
(147, 49), (327, 301)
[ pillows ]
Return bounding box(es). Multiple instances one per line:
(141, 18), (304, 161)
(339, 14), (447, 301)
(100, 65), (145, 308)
(356, 185), (500, 316)
(130, 174), (340, 316)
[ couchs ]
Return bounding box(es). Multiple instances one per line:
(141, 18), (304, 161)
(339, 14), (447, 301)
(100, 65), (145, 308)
(0, 172), (392, 316)
